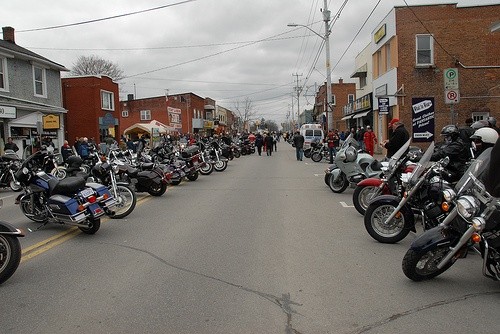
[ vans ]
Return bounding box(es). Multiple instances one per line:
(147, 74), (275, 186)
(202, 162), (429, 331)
(300, 124), (324, 148)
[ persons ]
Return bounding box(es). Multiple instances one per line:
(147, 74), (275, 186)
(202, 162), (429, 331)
(323, 125), (378, 164)
(457, 117), (499, 159)
(380, 119), (411, 163)
(293, 131), (305, 161)
(61, 140), (73, 161)
(100, 140), (109, 156)
(74, 136), (94, 159)
(4, 137), (19, 152)
(408, 124), (470, 183)
(106, 132), (293, 156)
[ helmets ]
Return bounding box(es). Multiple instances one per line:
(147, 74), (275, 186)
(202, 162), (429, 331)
(66, 155), (81, 168)
(479, 120), (489, 127)
(475, 128), (498, 146)
(343, 146), (357, 162)
(440, 125), (458, 134)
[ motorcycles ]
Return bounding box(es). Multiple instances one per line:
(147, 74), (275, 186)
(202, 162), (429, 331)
(401, 145), (500, 286)
(0, 110), (426, 236)
(363, 141), (466, 244)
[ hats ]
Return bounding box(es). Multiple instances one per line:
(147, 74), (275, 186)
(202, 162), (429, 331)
(84, 137), (88, 141)
(388, 119), (399, 127)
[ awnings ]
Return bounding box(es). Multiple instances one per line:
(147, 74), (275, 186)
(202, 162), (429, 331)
(341, 113), (355, 120)
(352, 109), (372, 119)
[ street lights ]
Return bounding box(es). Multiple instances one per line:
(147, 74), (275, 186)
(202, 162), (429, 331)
(288, 23), (335, 164)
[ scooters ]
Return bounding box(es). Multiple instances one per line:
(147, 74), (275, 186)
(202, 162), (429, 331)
(0, 220), (26, 284)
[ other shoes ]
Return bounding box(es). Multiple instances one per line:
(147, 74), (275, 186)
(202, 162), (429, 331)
(328, 162), (333, 164)
